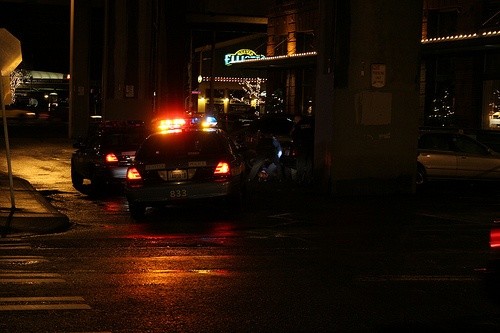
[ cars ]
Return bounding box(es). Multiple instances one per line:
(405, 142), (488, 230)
(71, 114), (315, 222)
(0, 103), (37, 121)
(417, 130), (500, 184)
